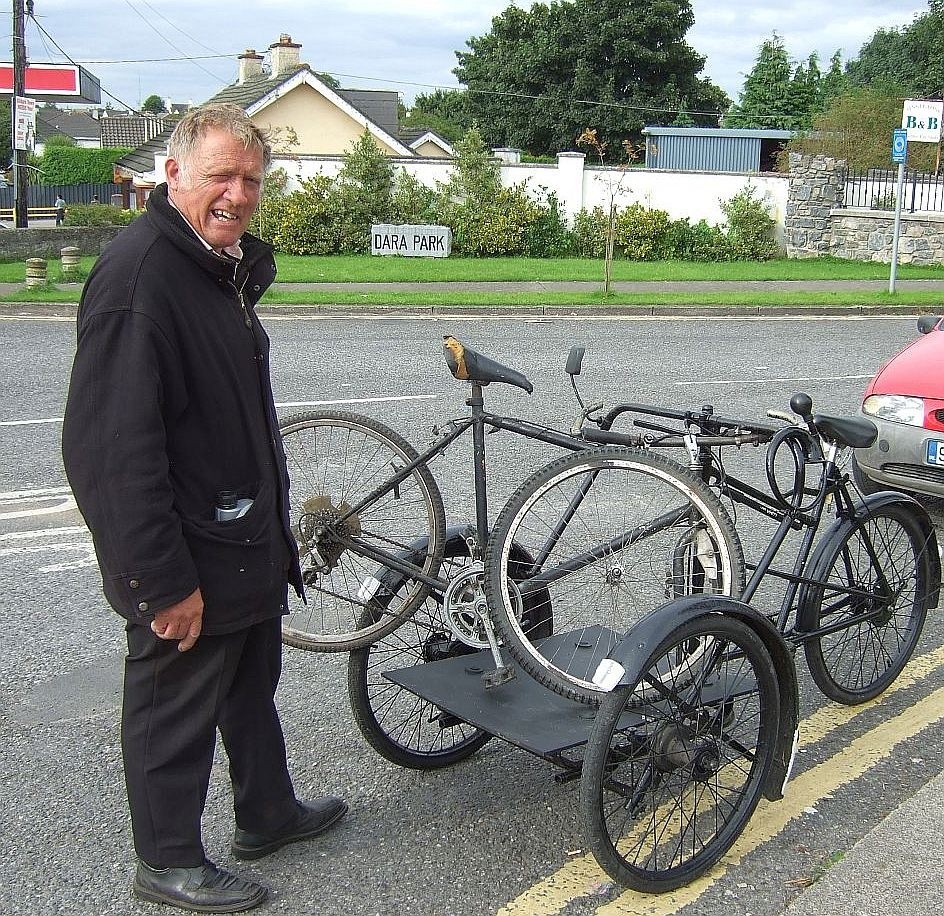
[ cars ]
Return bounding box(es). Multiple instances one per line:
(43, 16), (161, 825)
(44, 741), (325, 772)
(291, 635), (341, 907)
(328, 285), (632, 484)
(850, 311), (944, 500)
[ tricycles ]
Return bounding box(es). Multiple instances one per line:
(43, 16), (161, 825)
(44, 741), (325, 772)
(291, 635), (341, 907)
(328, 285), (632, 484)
(345, 346), (942, 899)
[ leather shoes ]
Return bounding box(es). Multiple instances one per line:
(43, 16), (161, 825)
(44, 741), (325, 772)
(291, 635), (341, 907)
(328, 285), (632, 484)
(231, 796), (348, 860)
(133, 857), (267, 912)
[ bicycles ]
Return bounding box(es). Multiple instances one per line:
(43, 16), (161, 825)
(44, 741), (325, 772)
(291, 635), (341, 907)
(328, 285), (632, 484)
(272, 333), (747, 710)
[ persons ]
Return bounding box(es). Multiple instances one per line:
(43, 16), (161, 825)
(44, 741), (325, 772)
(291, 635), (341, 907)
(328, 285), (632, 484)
(55, 194), (66, 226)
(62, 102), (348, 913)
(92, 194), (99, 203)
